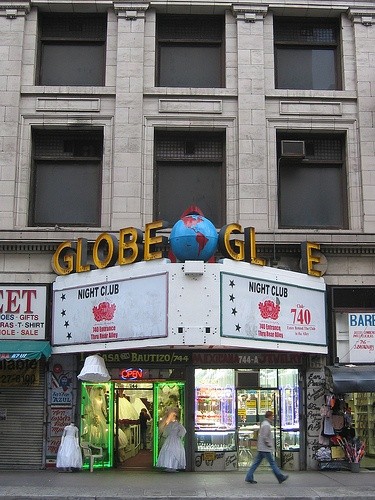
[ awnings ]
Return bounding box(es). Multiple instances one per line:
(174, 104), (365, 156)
(0, 340), (51, 360)
(324, 364), (375, 394)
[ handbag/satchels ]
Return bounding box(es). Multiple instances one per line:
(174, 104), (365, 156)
(321, 394), (344, 437)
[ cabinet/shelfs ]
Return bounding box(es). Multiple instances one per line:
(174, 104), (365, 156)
(354, 392), (375, 455)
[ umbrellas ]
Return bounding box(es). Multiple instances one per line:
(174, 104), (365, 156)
(354, 442), (365, 462)
(337, 437), (354, 463)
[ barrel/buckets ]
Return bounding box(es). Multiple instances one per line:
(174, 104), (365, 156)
(351, 463), (360, 473)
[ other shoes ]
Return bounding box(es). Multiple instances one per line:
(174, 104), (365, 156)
(245, 479), (257, 484)
(279, 475), (289, 484)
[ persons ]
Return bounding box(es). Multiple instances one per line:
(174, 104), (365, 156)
(137, 408), (150, 451)
(244, 411), (288, 484)
(157, 420), (186, 472)
(91, 388), (107, 424)
(56, 422), (82, 472)
